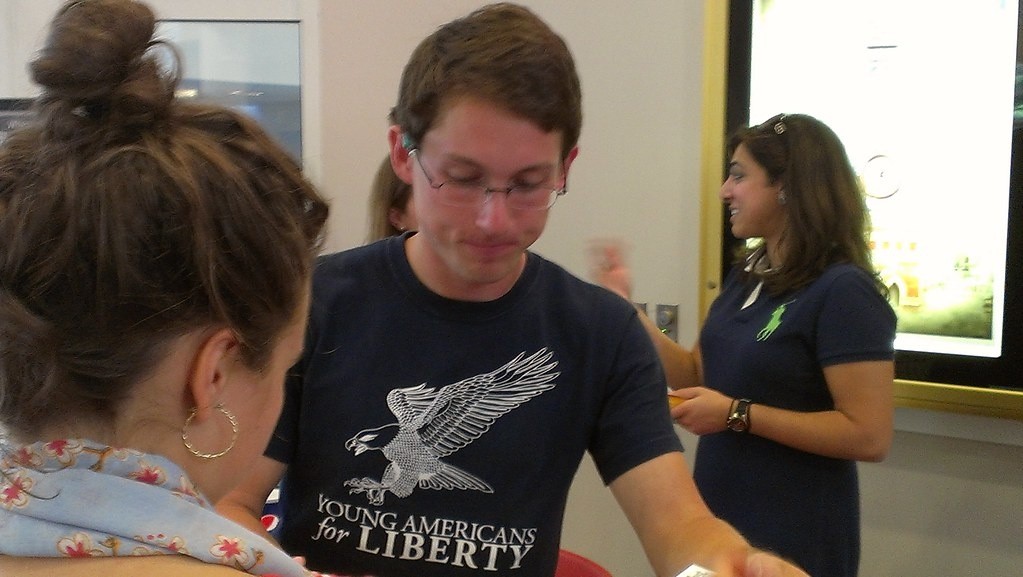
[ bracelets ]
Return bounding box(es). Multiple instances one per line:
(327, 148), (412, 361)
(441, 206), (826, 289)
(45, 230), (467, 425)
(747, 402), (755, 430)
(728, 399), (735, 421)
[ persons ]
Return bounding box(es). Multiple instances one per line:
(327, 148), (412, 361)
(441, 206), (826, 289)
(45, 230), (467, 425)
(0, 0), (335, 577)
(214, 1), (809, 577)
(368, 155), (417, 242)
(606, 114), (897, 577)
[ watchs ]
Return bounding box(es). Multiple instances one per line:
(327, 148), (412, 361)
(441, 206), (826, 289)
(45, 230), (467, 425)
(726, 398), (752, 432)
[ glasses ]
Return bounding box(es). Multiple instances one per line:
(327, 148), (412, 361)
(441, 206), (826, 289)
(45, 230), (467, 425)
(403, 138), (566, 211)
(756, 112), (790, 156)
